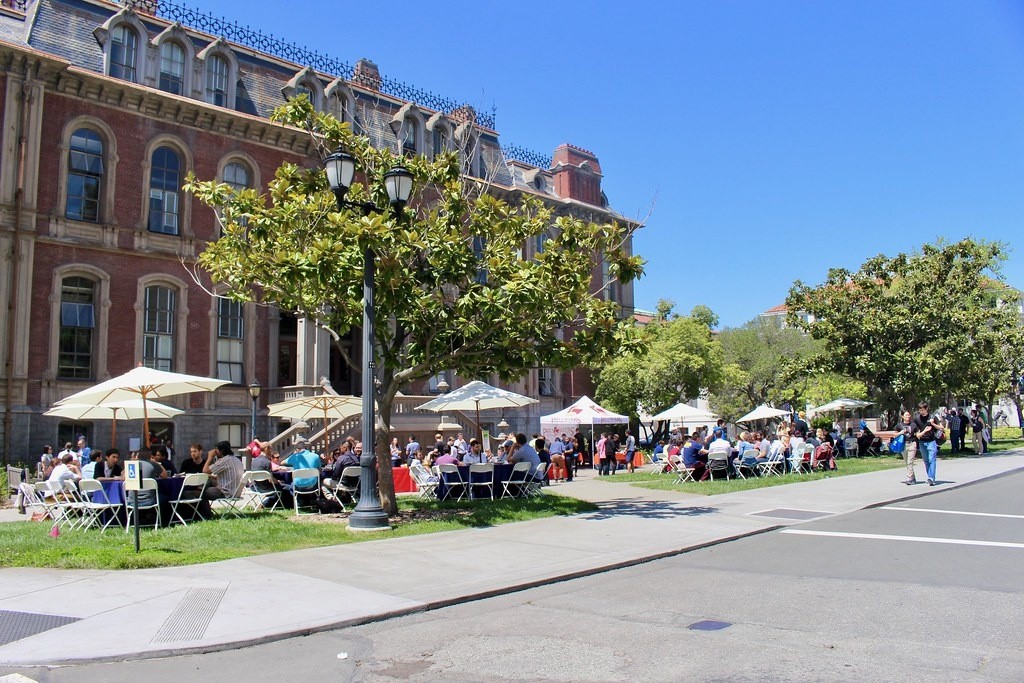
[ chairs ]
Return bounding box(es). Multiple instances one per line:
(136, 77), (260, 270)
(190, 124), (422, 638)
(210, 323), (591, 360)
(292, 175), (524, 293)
(409, 462), (553, 502)
(647, 438), (879, 484)
(20, 467), (362, 534)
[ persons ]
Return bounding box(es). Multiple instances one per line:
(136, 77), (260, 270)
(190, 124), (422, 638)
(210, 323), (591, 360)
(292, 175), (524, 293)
(893, 410), (932, 485)
(653, 411), (875, 483)
(914, 401), (944, 486)
(41, 436), (244, 529)
(549, 428), (635, 483)
(935, 404), (990, 455)
(246, 435), (403, 514)
(405, 433), (551, 501)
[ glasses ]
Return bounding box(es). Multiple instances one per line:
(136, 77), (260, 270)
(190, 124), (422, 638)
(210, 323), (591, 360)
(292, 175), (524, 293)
(48, 448), (52, 450)
(273, 457), (280, 460)
(796, 432), (800, 434)
(190, 450), (199, 453)
(334, 452), (340, 455)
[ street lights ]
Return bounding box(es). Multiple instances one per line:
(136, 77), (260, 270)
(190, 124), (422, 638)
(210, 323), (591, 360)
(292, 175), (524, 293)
(324, 144), (415, 535)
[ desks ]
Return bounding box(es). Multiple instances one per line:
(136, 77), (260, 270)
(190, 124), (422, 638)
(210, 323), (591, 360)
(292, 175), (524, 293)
(437, 464), (514, 500)
(392, 467), (417, 492)
(549, 458), (568, 478)
(93, 477), (196, 525)
(272, 470), (333, 507)
(594, 452), (643, 467)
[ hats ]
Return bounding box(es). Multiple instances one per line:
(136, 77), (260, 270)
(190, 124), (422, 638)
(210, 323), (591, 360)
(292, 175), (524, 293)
(671, 426), (677, 431)
(614, 434), (620, 438)
(505, 440), (514, 448)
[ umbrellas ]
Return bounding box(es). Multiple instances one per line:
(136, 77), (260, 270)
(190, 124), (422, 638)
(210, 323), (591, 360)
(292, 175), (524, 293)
(53, 361), (232, 449)
(810, 398), (877, 428)
(735, 406), (792, 433)
(267, 390), (378, 458)
(413, 380), (540, 440)
(651, 403), (717, 441)
(42, 399), (185, 449)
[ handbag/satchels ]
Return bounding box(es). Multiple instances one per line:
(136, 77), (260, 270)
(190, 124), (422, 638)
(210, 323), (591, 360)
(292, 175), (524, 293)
(981, 429), (990, 444)
(889, 434), (905, 454)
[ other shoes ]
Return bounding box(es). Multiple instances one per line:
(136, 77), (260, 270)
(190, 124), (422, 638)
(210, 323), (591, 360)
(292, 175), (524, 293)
(258, 505), (264, 510)
(690, 479), (694, 482)
(699, 480), (706, 482)
(558, 480), (567, 483)
(927, 477), (936, 486)
(901, 478), (917, 486)
(554, 479), (558, 483)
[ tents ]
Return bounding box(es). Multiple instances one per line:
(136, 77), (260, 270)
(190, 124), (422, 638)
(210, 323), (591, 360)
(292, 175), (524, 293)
(540, 395), (629, 470)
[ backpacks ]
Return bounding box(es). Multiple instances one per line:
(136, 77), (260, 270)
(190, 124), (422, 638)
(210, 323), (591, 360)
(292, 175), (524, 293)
(930, 414), (947, 447)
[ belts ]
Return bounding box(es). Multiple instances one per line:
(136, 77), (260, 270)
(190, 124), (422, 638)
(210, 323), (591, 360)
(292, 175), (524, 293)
(552, 452), (562, 455)
